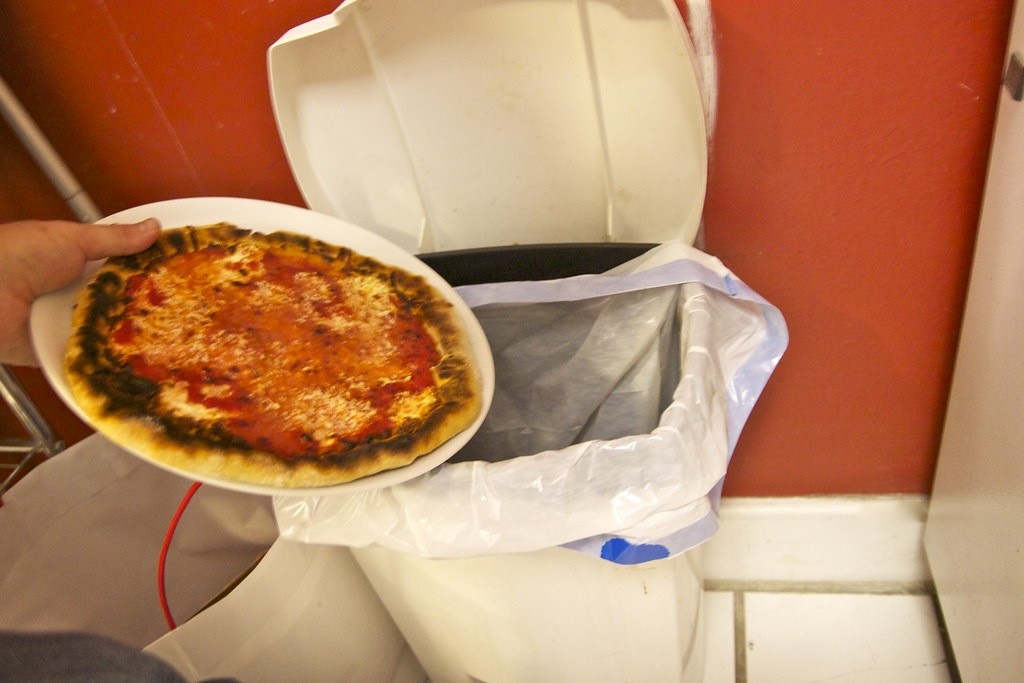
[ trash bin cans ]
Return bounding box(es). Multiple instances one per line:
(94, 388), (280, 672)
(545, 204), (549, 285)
(270, 1), (718, 683)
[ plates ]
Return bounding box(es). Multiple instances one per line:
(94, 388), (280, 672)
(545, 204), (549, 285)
(29, 197), (496, 498)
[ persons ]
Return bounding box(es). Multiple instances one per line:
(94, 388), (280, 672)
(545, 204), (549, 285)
(1, 216), (237, 683)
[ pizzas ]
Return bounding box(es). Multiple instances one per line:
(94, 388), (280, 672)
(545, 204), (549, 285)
(62, 221), (482, 489)
